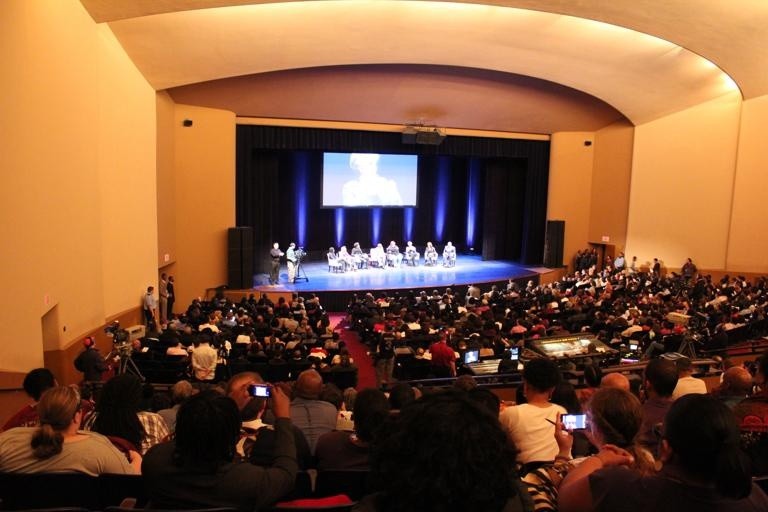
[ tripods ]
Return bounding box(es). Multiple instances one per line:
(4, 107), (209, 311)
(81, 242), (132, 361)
(117, 357), (147, 381)
(293, 263), (309, 283)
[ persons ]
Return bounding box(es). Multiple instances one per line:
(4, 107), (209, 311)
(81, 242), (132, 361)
(337, 154), (403, 205)
(0, 237), (767, 512)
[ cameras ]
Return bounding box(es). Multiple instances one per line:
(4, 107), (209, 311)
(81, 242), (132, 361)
(629, 344), (638, 351)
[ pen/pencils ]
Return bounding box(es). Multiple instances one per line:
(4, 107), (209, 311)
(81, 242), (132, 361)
(543, 417), (574, 435)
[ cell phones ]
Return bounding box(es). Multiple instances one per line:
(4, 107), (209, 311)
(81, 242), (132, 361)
(247, 383), (272, 399)
(560, 413), (587, 431)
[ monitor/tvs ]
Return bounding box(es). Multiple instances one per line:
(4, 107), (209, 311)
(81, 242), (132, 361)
(503, 345), (521, 361)
(464, 349), (480, 365)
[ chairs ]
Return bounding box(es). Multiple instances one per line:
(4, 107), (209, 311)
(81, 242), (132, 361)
(326, 246), (456, 274)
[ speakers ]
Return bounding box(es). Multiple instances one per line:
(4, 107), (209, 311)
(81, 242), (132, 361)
(544, 218), (565, 269)
(226, 226), (256, 289)
